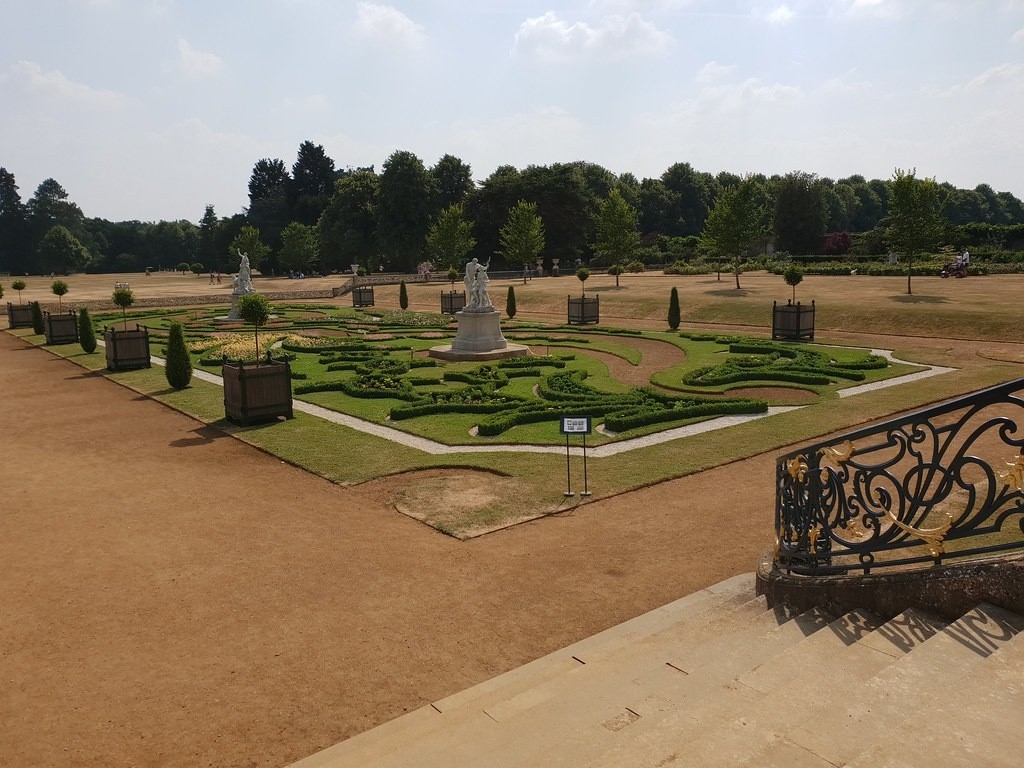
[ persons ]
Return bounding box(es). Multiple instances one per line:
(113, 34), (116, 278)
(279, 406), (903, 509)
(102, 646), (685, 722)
(956, 247), (969, 278)
(237, 248), (253, 292)
(233, 276), (239, 292)
(115, 281), (129, 290)
(462, 258), (493, 308)
(209, 271), (222, 285)
(888, 249), (897, 266)
(289, 268), (305, 279)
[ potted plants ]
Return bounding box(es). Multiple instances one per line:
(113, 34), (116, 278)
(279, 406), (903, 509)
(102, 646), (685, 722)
(7, 280), (34, 329)
(772, 266), (815, 340)
(222, 290), (294, 428)
(352, 267), (374, 308)
(43, 280), (79, 346)
(567, 268), (599, 325)
(103, 287), (150, 373)
(440, 269), (466, 315)
